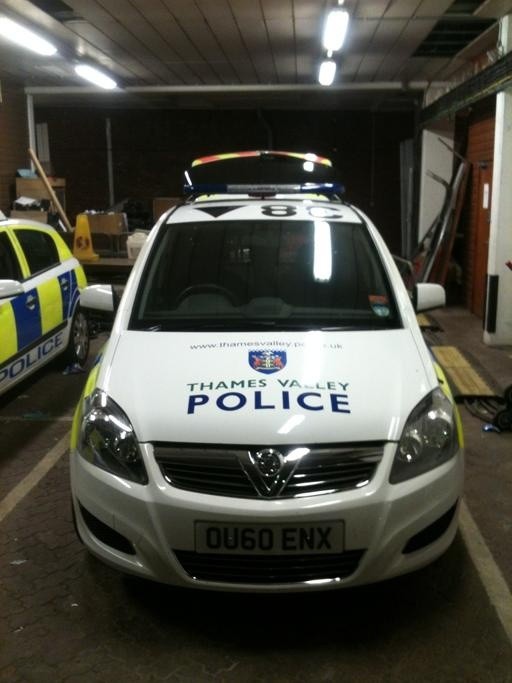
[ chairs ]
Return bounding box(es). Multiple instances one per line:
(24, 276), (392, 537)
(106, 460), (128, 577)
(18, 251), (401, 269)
(176, 226), (348, 305)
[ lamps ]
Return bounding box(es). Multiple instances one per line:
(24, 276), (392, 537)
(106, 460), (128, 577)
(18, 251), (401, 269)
(316, 9), (349, 87)
(0, 12), (120, 93)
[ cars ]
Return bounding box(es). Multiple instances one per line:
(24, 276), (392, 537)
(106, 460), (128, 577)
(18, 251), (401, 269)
(0, 208), (92, 399)
(64, 191), (468, 599)
